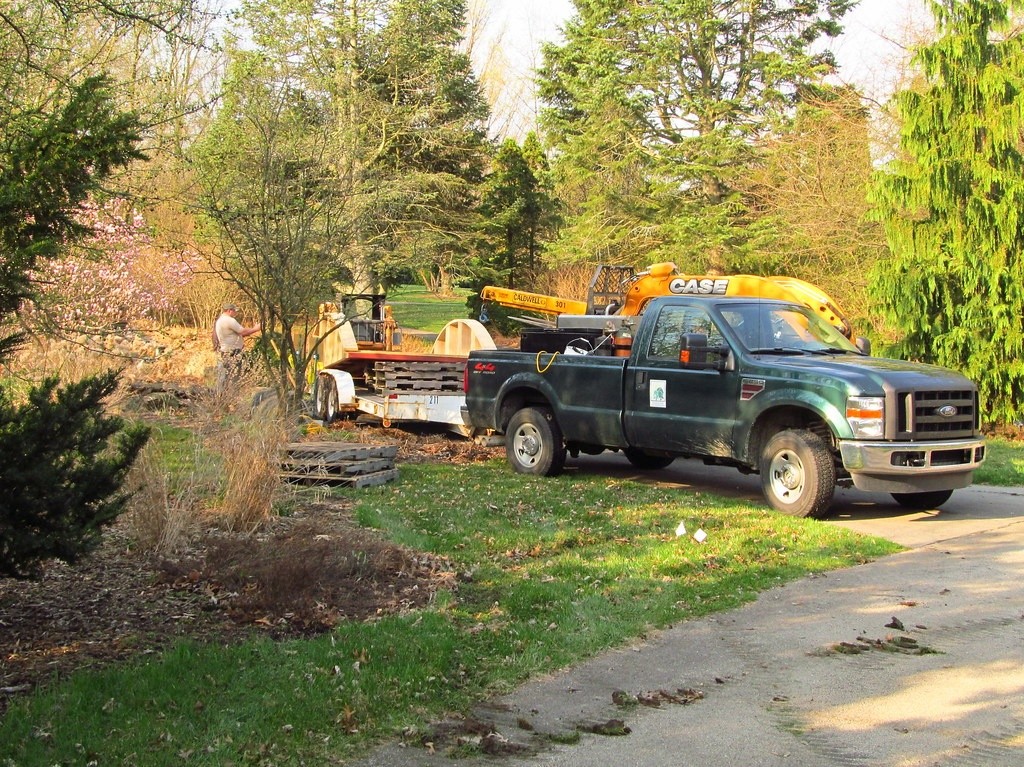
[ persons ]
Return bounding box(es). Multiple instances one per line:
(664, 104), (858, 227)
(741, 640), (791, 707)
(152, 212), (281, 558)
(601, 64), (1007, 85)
(212, 312), (224, 397)
(215, 303), (262, 400)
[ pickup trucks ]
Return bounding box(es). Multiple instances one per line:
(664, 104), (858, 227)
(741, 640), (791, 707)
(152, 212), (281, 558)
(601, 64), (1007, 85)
(460, 295), (987, 519)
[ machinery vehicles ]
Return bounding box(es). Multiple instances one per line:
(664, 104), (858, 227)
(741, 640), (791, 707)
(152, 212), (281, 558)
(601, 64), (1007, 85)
(306, 289), (402, 396)
(477, 261), (856, 356)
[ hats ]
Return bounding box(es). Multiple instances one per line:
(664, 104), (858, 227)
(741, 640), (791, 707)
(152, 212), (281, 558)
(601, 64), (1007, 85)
(224, 304), (240, 309)
